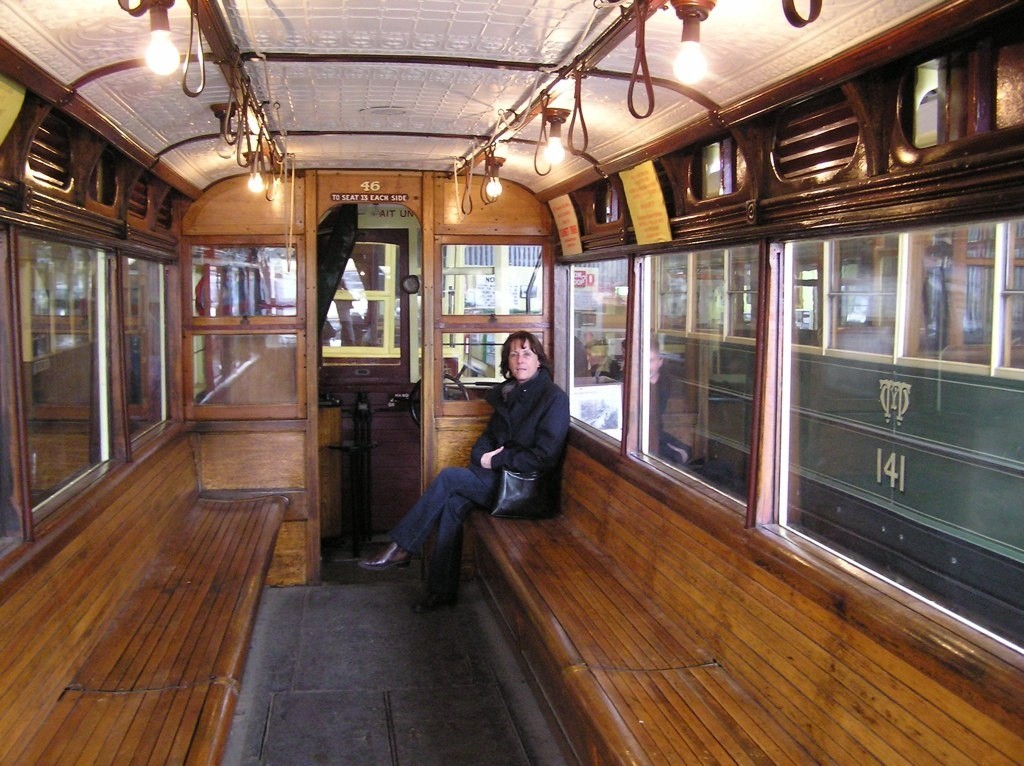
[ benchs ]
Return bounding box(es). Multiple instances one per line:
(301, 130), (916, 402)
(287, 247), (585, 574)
(465, 439), (1022, 766)
(0, 419), (289, 765)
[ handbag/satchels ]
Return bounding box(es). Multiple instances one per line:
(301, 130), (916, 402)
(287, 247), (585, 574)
(490, 467), (562, 520)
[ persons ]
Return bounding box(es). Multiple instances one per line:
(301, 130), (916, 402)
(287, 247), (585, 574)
(349, 330), (571, 615)
(646, 338), (696, 467)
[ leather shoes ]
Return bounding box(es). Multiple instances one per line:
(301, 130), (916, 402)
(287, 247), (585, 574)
(411, 588), (458, 613)
(358, 542), (411, 571)
